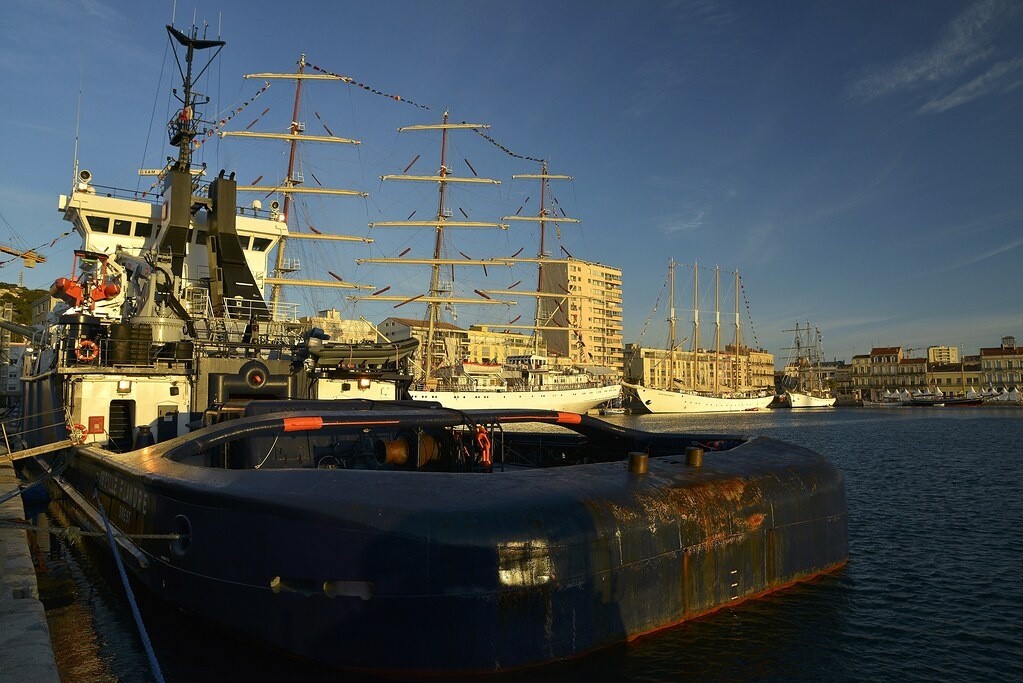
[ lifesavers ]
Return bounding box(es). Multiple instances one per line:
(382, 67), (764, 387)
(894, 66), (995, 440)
(74, 340), (98, 361)
(68, 424), (88, 444)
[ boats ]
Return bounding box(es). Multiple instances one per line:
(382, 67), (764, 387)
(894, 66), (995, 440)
(861, 399), (903, 407)
(18, 0), (854, 683)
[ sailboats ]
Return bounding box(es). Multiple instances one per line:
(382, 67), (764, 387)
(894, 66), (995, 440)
(225, 53), (400, 404)
(622, 256), (776, 414)
(778, 319), (837, 408)
(345, 107), (623, 417)
(901, 339), (1004, 407)
